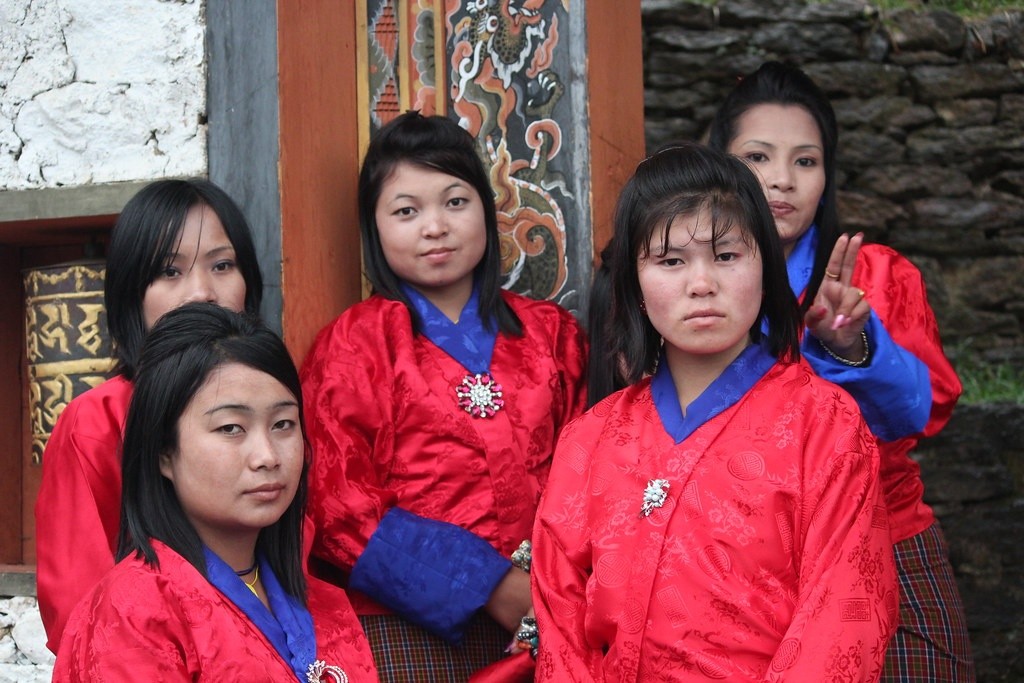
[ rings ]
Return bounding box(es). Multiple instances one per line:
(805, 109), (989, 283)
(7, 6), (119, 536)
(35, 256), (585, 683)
(824, 267), (840, 278)
(853, 286), (865, 298)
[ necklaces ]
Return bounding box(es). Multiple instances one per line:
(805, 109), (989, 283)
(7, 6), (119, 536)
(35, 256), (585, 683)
(237, 564), (259, 598)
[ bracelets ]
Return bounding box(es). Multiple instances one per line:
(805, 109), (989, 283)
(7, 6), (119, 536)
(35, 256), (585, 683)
(813, 329), (870, 367)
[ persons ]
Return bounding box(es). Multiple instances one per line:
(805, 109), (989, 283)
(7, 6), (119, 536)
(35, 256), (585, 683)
(48, 302), (380, 682)
(296, 107), (590, 682)
(699, 58), (976, 683)
(528, 142), (903, 683)
(36, 175), (265, 652)
